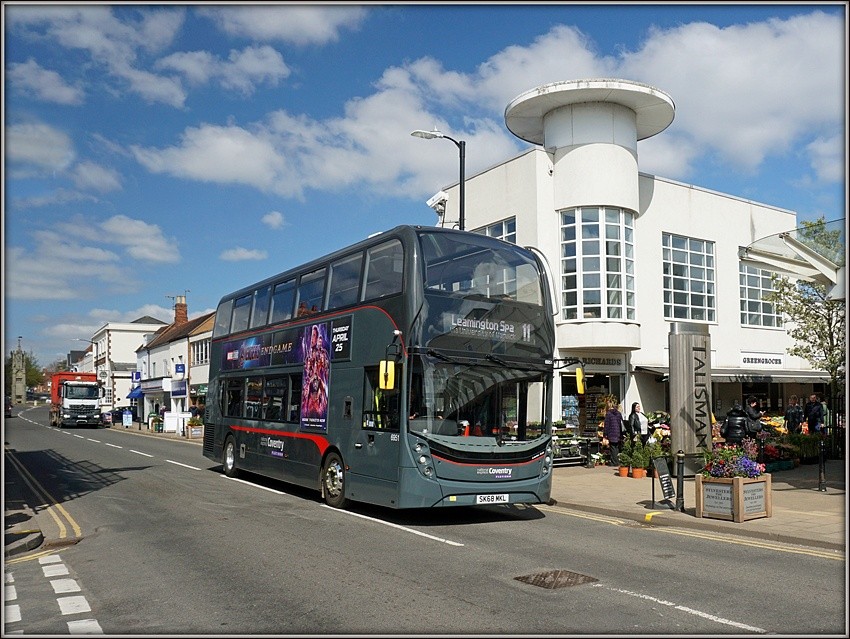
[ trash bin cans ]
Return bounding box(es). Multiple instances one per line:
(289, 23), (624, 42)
(123, 410), (132, 428)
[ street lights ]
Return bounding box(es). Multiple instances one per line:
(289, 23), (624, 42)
(72, 337), (98, 373)
(410, 125), (466, 232)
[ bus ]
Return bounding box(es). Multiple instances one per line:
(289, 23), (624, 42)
(203, 224), (589, 510)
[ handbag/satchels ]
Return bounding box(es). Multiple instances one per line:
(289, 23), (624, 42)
(720, 416), (728, 438)
(621, 419), (627, 432)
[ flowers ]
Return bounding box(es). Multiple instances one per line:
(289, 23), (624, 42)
(703, 429), (799, 478)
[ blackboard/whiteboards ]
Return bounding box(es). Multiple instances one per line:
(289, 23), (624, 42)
(652, 456), (675, 499)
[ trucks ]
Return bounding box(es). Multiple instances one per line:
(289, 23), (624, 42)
(49, 371), (105, 428)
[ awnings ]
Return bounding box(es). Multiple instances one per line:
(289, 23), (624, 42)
(127, 386), (144, 399)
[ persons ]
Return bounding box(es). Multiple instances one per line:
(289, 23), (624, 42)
(409, 388), (420, 420)
(784, 395), (803, 434)
(298, 324), (329, 415)
(804, 395), (829, 434)
(188, 402), (206, 417)
(297, 301), (318, 317)
(720, 395), (766, 463)
(629, 403), (652, 445)
(603, 400), (623, 466)
(636, 364), (847, 384)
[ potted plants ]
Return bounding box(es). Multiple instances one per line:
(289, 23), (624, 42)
(595, 434), (668, 478)
(508, 420), (581, 458)
(186, 416), (204, 439)
(150, 417), (161, 432)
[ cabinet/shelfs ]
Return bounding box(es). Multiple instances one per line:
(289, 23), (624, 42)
(552, 425), (586, 466)
(581, 388), (607, 436)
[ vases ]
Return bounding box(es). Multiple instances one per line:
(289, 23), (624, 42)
(765, 463), (779, 473)
(696, 472), (772, 522)
(779, 460), (794, 470)
